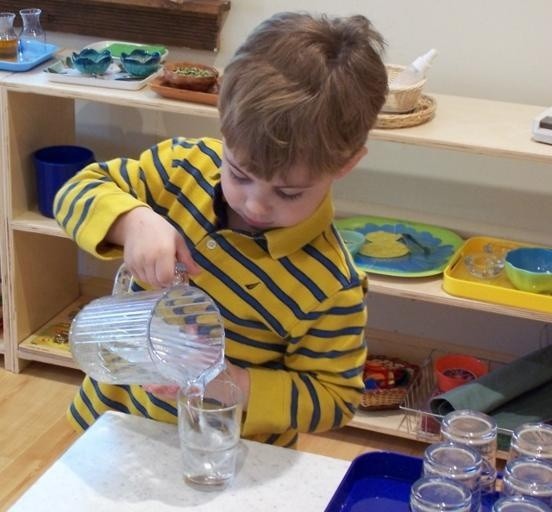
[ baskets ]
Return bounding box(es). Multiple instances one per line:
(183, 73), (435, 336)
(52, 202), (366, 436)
(361, 355), (421, 411)
(399, 348), (514, 451)
(381, 64), (427, 113)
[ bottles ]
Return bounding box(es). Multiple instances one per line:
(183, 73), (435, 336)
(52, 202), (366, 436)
(1, 7), (46, 62)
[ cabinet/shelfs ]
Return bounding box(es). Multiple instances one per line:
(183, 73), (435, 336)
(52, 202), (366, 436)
(2, 50), (552, 461)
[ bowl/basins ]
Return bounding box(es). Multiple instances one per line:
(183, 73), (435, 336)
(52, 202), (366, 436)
(70, 45), (161, 78)
(457, 245), (510, 276)
(164, 62), (226, 92)
(503, 247), (552, 295)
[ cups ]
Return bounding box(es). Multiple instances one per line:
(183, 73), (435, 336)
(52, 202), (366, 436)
(68, 258), (229, 393)
(339, 229), (367, 257)
(410, 409), (551, 512)
(174, 380), (247, 492)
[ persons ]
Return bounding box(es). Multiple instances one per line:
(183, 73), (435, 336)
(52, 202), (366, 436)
(49, 9), (389, 459)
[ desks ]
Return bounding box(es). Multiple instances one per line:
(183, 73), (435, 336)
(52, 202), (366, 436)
(7, 410), (350, 512)
